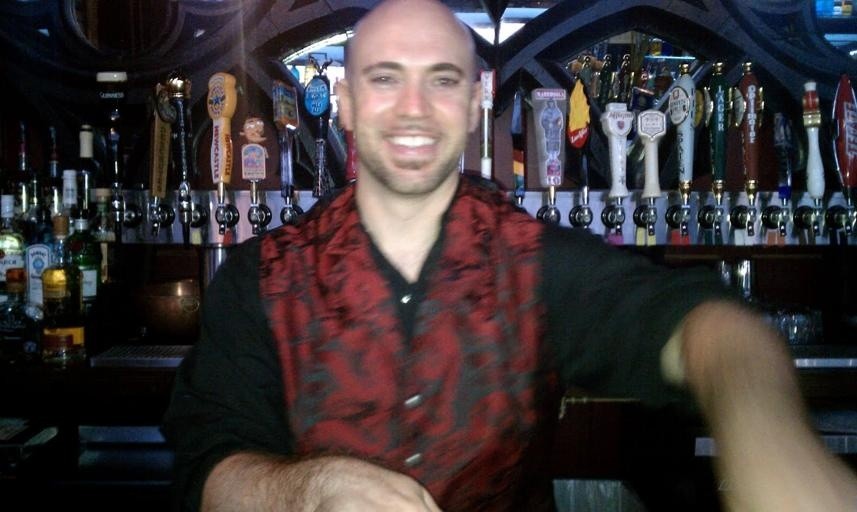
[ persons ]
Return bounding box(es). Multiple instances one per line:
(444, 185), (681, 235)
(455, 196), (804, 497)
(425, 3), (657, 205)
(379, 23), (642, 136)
(155, 1), (856, 512)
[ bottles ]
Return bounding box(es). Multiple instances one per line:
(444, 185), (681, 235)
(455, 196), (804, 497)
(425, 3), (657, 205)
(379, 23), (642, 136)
(0, 118), (101, 363)
